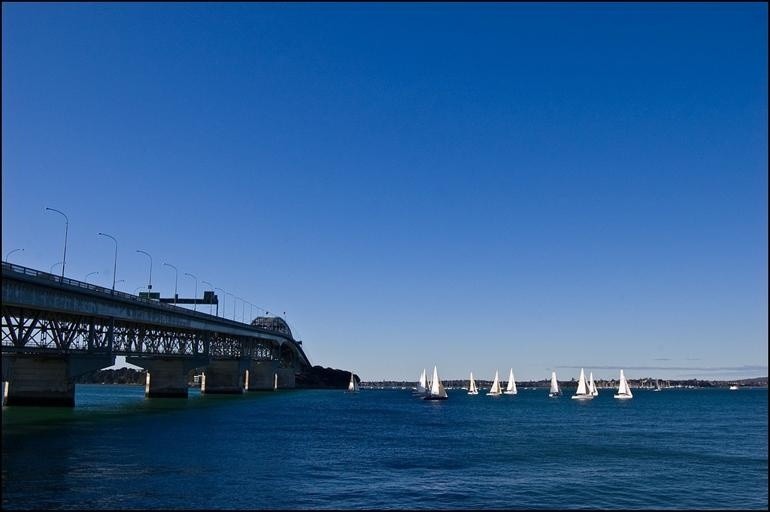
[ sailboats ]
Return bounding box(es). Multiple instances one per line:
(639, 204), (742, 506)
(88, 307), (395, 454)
(730, 385), (738, 391)
(344, 373), (360, 393)
(412, 365), (633, 400)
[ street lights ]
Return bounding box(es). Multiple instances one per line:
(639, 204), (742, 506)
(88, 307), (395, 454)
(6, 207), (273, 331)
